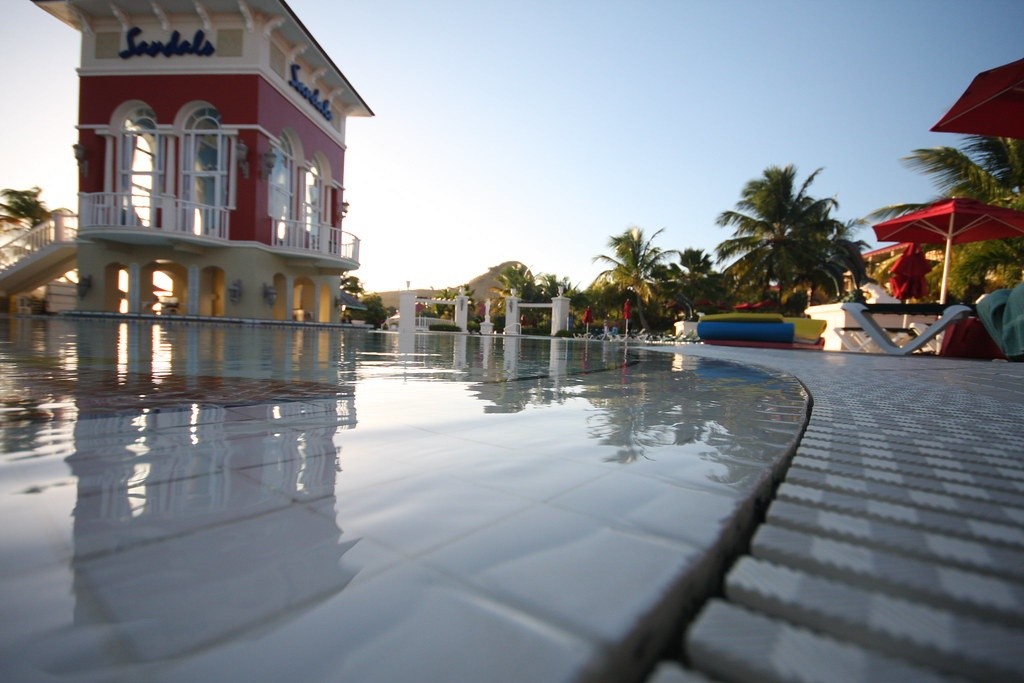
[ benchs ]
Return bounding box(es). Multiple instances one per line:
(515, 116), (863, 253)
(832, 326), (914, 354)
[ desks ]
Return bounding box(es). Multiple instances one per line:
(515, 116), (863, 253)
(837, 301), (975, 357)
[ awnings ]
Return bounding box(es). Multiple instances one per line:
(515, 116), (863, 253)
(339, 291), (367, 310)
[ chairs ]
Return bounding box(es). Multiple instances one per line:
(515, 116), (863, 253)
(573, 326), (705, 346)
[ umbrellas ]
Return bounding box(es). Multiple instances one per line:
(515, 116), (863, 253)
(890, 244), (931, 330)
(735, 301), (752, 309)
(583, 308), (592, 334)
(621, 343), (628, 384)
(873, 197), (1024, 319)
(581, 339), (590, 374)
(521, 317), (527, 327)
(929, 58), (1024, 140)
(624, 300), (632, 337)
(753, 298), (778, 309)
(666, 299), (731, 316)
